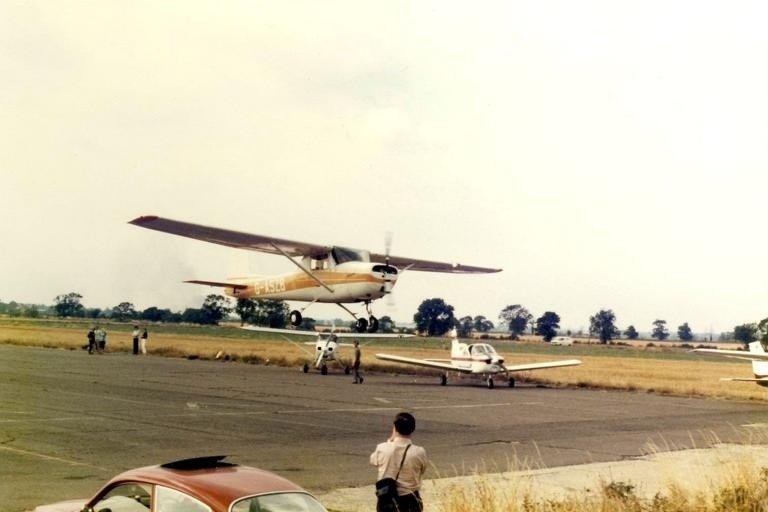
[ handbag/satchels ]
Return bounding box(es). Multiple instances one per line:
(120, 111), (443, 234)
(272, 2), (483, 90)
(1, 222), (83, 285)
(376, 477), (398, 504)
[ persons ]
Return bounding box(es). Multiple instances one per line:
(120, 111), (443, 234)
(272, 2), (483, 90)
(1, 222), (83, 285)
(369, 412), (430, 512)
(141, 327), (148, 355)
(87, 325), (108, 355)
(351, 339), (363, 384)
(131, 325), (141, 354)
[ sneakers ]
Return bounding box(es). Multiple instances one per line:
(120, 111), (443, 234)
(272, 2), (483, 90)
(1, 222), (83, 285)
(353, 379), (363, 384)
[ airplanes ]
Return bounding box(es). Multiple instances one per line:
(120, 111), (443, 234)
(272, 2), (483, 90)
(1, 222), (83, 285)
(685, 340), (768, 391)
(237, 325), (417, 385)
(374, 337), (584, 389)
(128, 212), (504, 333)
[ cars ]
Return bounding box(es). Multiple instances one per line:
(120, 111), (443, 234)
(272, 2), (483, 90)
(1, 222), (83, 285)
(34, 453), (333, 512)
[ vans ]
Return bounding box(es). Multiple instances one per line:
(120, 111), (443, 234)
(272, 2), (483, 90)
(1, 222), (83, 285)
(551, 336), (572, 347)
